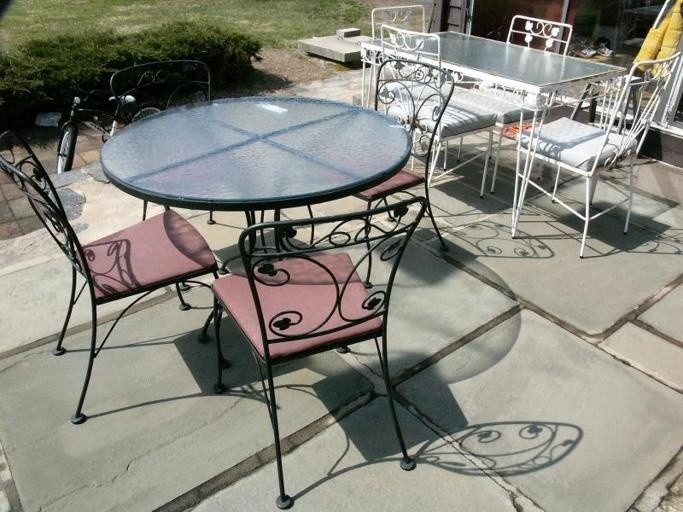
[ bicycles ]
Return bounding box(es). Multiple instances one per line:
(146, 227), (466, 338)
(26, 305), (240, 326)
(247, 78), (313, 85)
(56, 94), (166, 175)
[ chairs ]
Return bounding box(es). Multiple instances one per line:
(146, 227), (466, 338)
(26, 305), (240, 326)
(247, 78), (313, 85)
(508, 69), (666, 257)
(194, 196), (427, 510)
(350, 56), (456, 247)
(482, 15), (573, 198)
(603, 50), (682, 180)
(378, 25), (499, 197)
(369, 6), (427, 65)
(0, 133), (225, 424)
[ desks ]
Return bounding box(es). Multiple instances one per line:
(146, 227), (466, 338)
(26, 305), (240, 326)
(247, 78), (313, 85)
(107, 94), (414, 273)
(360, 29), (627, 239)
